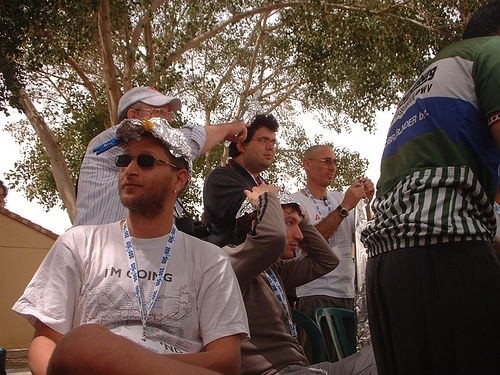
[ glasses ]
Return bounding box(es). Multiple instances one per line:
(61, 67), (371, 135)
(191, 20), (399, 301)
(115, 155), (177, 170)
(308, 158), (338, 165)
(251, 138), (277, 148)
(135, 107), (176, 121)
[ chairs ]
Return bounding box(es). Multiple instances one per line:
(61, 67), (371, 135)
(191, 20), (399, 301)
(291, 308), (357, 366)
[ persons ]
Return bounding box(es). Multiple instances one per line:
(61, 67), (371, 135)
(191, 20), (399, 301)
(362, 4), (500, 375)
(67, 85), (251, 240)
(219, 183), (378, 375)
(12, 116), (252, 375)
(282, 145), (375, 364)
(203, 113), (279, 258)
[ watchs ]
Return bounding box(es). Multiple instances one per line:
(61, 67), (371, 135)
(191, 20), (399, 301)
(336, 204), (350, 218)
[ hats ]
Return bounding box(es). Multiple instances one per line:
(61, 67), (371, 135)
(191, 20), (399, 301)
(236, 188), (298, 219)
(115, 115), (193, 183)
(118, 87), (182, 120)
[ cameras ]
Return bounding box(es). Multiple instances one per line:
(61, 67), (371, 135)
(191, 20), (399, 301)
(177, 216), (212, 240)
